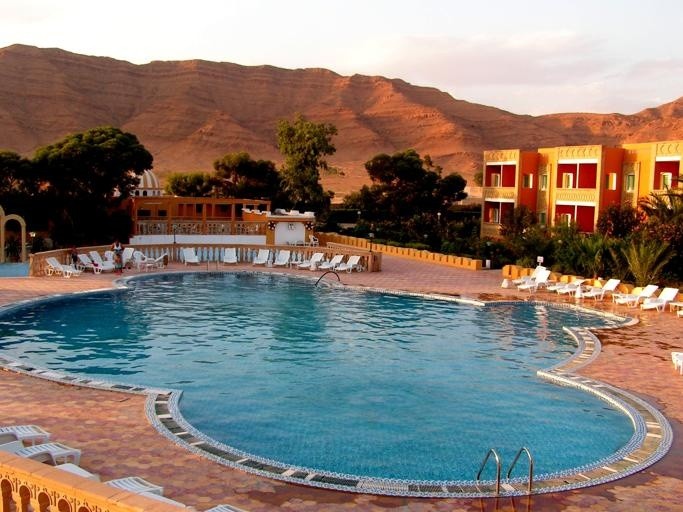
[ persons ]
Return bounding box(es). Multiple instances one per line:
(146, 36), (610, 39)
(110, 238), (125, 266)
(71, 247), (79, 266)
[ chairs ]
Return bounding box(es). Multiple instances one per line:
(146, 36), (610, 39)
(252, 248), (363, 273)
(512, 265), (680, 313)
(223, 248), (238, 267)
(44, 248), (167, 278)
(1, 421), (244, 511)
(182, 247), (200, 266)
(309, 235), (319, 246)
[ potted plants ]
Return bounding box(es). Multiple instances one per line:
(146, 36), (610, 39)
(4, 235), (22, 263)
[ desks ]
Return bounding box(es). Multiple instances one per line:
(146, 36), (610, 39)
(669, 301), (683, 313)
